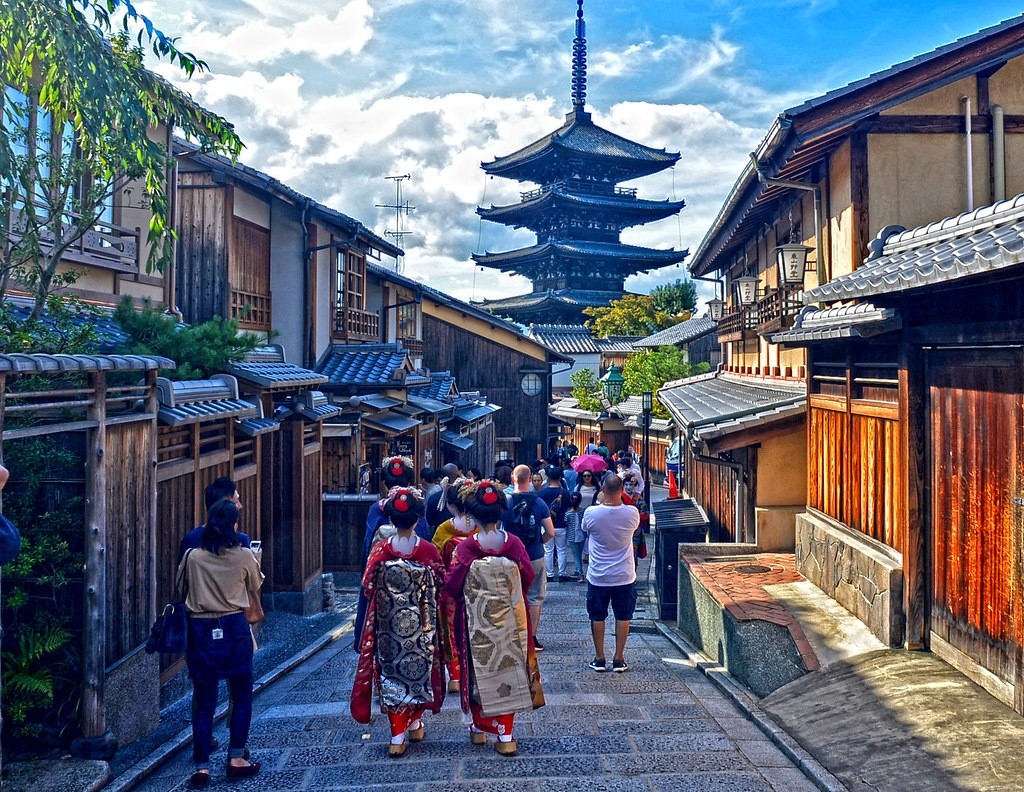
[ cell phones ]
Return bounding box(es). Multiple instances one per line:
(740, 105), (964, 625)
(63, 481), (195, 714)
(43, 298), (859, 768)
(249, 540), (261, 551)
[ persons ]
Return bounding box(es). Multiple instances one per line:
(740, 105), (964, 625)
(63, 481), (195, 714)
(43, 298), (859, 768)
(179, 477), (250, 761)
(431, 483), (480, 692)
(434, 463), (482, 486)
(416, 467), (436, 498)
(174, 500), (265, 789)
(497, 463), (555, 652)
(445, 478), (545, 755)
(354, 455), (430, 655)
(581, 475), (641, 673)
(350, 486), (454, 757)
(495, 437), (653, 583)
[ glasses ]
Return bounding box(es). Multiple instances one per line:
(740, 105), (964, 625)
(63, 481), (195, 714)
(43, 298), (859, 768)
(582, 475), (591, 478)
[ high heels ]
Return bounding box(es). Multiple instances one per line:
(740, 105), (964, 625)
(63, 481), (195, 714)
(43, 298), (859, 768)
(389, 738), (406, 756)
(494, 735), (516, 754)
(470, 722), (486, 744)
(448, 680), (460, 692)
(408, 722), (424, 743)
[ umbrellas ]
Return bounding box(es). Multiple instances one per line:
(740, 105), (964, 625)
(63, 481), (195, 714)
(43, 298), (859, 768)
(571, 453), (609, 473)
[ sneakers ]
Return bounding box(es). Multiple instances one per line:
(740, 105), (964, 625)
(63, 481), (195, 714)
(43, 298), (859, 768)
(588, 658), (606, 671)
(613, 658), (628, 671)
(534, 639), (543, 651)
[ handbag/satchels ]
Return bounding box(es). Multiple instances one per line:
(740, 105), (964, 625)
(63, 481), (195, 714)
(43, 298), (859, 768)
(145, 548), (194, 654)
(547, 490), (563, 524)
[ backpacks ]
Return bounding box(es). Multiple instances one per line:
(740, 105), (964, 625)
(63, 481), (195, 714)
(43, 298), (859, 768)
(506, 493), (539, 548)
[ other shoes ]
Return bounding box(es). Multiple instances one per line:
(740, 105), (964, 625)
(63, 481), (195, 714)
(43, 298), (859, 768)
(226, 749), (249, 762)
(209, 736), (218, 754)
(577, 576), (584, 582)
(568, 574), (578, 578)
(558, 576), (570, 582)
(227, 760), (260, 780)
(547, 577), (554, 582)
(191, 773), (211, 788)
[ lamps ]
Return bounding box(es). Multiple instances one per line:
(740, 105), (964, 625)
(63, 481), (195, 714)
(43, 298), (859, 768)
(730, 245), (763, 306)
(705, 264), (727, 323)
(769, 187), (816, 287)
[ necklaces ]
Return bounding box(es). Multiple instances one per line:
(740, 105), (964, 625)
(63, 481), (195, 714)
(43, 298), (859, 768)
(604, 499), (621, 504)
